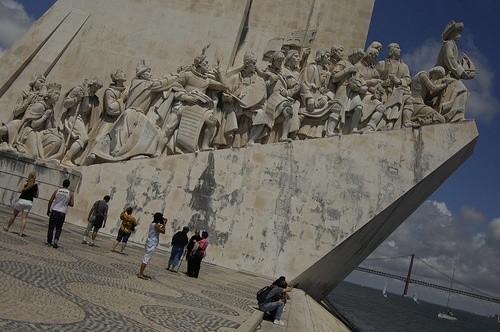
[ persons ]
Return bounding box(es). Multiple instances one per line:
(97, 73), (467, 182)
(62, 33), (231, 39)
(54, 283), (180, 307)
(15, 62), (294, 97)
(82, 195), (111, 245)
(260, 276), (293, 326)
(55, 77), (103, 166)
(47, 179), (74, 248)
(111, 206), (137, 254)
(186, 229), (209, 278)
(3, 172), (39, 236)
(166, 226), (189, 272)
(0, 72), (64, 163)
(75, 19), (470, 166)
(138, 212), (168, 280)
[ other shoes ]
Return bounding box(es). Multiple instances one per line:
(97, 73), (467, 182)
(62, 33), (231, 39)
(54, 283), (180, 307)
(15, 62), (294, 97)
(110, 248), (124, 254)
(138, 274), (148, 280)
(273, 319), (285, 326)
(82, 239), (87, 244)
(45, 241), (58, 248)
(166, 267), (176, 272)
(88, 242), (97, 247)
(183, 271), (198, 278)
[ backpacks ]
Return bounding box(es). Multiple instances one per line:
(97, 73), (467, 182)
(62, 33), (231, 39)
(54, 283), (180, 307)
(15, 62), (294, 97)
(257, 284), (275, 302)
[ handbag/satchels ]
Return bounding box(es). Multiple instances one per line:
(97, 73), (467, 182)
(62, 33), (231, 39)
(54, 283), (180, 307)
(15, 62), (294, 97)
(88, 209), (98, 223)
